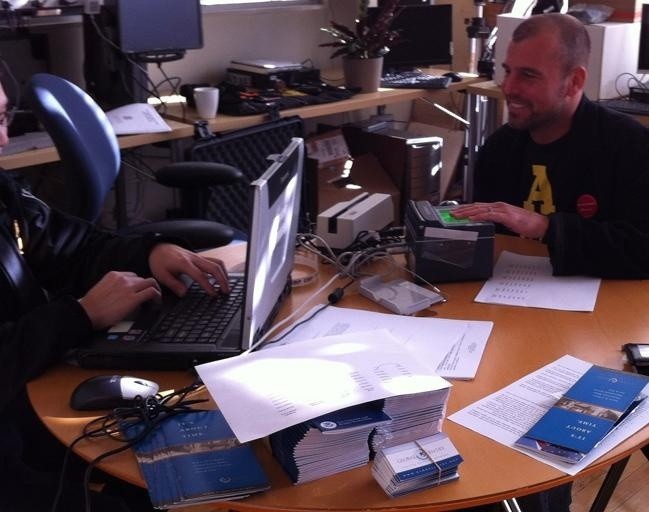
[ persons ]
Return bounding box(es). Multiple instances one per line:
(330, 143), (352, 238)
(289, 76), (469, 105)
(0, 58), (230, 511)
(447, 12), (648, 281)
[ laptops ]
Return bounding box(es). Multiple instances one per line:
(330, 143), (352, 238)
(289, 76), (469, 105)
(86, 135), (305, 371)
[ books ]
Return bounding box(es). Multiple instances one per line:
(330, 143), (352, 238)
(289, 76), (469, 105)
(229, 63), (303, 77)
(230, 58), (301, 70)
(513, 390), (649, 465)
(117, 386), (454, 510)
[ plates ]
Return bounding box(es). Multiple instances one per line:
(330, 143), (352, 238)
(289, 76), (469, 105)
(289, 264), (318, 288)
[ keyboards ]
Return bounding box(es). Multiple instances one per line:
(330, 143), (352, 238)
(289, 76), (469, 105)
(380, 74), (452, 89)
(599, 99), (649, 116)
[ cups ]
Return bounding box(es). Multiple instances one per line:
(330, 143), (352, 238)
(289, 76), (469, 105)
(193, 87), (219, 118)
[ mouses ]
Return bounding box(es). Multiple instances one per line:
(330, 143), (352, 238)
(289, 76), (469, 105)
(69, 375), (159, 411)
(443, 72), (463, 82)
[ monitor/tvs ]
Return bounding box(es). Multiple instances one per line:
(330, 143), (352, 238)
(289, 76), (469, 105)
(116, 0), (203, 54)
(366, 4), (453, 71)
(636, 3), (649, 74)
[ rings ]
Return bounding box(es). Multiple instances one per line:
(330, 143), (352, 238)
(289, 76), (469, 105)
(488, 206), (494, 213)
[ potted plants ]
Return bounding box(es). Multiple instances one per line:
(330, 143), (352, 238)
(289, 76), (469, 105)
(317, 8), (397, 91)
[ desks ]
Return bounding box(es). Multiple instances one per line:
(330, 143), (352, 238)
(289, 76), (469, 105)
(26, 229), (649, 511)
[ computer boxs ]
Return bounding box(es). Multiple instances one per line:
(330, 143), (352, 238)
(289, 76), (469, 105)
(339, 121), (443, 205)
